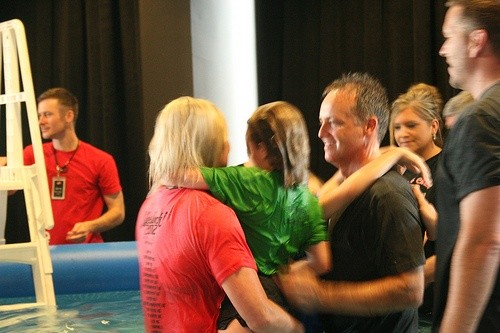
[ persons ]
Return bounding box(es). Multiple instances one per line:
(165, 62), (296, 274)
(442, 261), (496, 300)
(135, 0), (500, 333)
(0, 88), (126, 246)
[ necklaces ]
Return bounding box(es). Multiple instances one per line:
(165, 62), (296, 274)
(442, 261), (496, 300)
(52, 144), (79, 178)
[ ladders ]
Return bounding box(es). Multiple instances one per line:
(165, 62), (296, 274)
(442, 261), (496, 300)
(1, 18), (58, 316)
